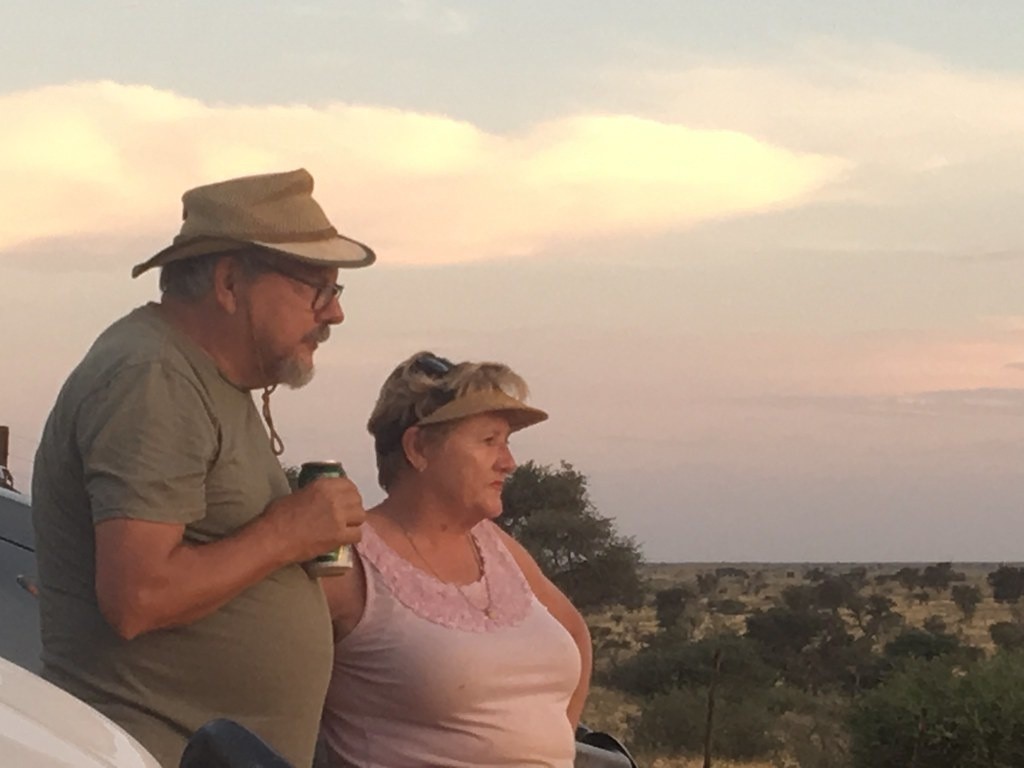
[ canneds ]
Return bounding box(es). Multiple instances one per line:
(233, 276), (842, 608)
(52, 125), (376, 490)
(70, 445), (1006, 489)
(298, 460), (353, 577)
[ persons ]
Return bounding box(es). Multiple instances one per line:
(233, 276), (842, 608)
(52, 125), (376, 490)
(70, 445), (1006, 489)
(314, 351), (592, 768)
(31, 170), (377, 768)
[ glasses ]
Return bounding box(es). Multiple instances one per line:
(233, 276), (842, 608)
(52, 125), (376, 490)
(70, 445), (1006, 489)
(416, 355), (448, 380)
(240, 257), (343, 314)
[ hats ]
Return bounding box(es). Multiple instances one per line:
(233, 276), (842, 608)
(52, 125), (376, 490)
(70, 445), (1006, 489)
(410, 386), (548, 433)
(132, 169), (375, 279)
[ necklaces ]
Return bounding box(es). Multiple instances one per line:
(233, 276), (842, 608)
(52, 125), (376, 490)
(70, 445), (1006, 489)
(392, 512), (495, 621)
